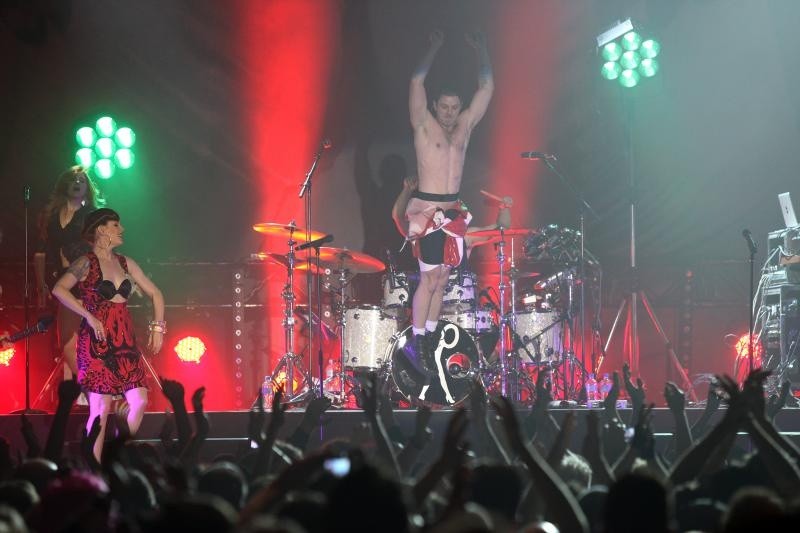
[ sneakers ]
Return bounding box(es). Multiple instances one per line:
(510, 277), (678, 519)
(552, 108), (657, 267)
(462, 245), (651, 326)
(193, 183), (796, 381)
(424, 329), (438, 370)
(396, 331), (435, 385)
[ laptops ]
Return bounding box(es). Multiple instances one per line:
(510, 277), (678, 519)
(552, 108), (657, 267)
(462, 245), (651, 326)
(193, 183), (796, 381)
(778, 192), (800, 228)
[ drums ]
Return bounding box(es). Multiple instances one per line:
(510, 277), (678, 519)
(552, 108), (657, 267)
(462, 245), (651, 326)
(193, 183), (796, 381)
(387, 321), (483, 410)
(508, 310), (567, 364)
(442, 268), (479, 302)
(439, 311), (495, 330)
(342, 304), (400, 371)
(383, 272), (413, 306)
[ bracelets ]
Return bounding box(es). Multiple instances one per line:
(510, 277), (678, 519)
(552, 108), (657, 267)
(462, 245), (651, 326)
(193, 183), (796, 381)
(149, 321), (167, 334)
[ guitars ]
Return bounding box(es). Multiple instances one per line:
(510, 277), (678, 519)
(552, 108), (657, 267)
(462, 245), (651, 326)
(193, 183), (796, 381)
(0, 315), (53, 350)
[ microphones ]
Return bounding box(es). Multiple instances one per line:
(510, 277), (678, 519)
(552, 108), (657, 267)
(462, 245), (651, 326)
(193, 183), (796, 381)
(23, 184), (31, 205)
(294, 234), (334, 251)
(6, 317), (52, 341)
(320, 138), (332, 148)
(742, 229), (757, 253)
(495, 207), (510, 228)
(520, 152), (556, 160)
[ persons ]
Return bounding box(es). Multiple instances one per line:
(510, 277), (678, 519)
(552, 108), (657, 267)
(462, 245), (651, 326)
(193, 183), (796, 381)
(408, 31), (494, 336)
(53, 208), (166, 459)
(0, 364), (800, 533)
(33, 166), (97, 381)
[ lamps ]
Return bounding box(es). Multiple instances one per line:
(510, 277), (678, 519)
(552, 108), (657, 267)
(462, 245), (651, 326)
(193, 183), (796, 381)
(596, 17), (663, 88)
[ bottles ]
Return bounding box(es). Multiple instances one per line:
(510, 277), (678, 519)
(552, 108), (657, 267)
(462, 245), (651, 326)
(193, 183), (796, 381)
(599, 373), (613, 400)
(262, 376), (273, 412)
(585, 373), (600, 408)
(326, 360), (336, 390)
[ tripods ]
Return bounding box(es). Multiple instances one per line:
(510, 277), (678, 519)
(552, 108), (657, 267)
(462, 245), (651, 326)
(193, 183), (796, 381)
(248, 229), (361, 410)
(492, 159), (601, 407)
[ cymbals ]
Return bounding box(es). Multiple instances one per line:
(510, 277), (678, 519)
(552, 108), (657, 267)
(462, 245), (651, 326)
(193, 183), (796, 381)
(291, 246), (385, 274)
(465, 226), (534, 237)
(494, 269), (544, 280)
(251, 251), (309, 271)
(253, 221), (333, 242)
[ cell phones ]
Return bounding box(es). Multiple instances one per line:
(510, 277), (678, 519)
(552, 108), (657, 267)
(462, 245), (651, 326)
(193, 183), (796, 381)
(322, 455), (352, 479)
